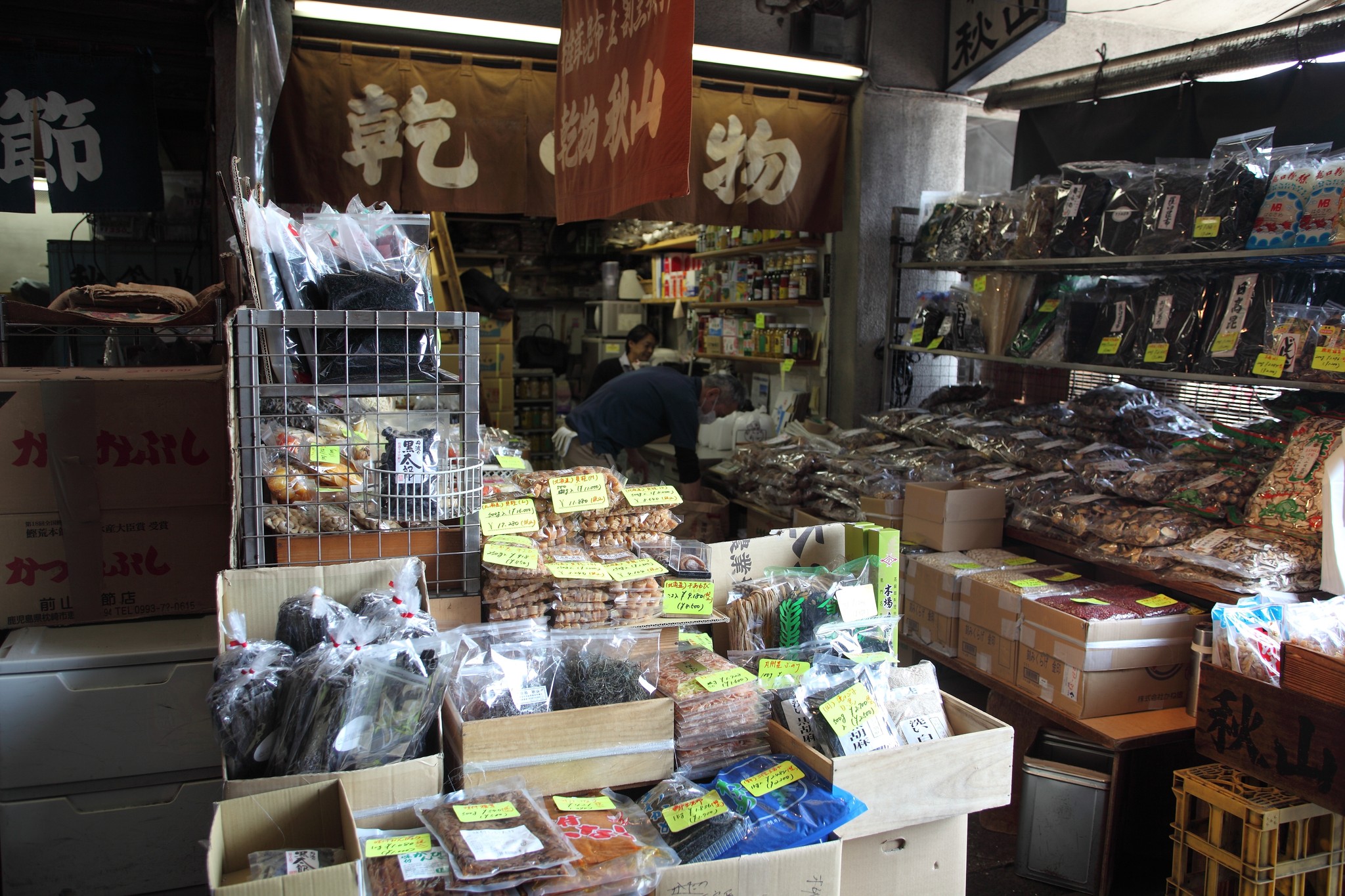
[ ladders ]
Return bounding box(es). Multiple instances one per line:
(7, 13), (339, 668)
(408, 210), (492, 429)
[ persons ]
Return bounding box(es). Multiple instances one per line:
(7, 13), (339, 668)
(560, 366), (743, 502)
(582, 324), (660, 403)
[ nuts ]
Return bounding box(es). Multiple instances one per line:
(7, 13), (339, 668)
(262, 491), (404, 536)
(351, 441), (372, 459)
(275, 459), (331, 487)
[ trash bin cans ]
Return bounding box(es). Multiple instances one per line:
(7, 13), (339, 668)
(1016, 726), (1123, 894)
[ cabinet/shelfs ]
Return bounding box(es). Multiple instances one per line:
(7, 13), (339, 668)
(500, 178), (1345, 474)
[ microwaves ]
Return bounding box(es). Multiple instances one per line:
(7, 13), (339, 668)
(584, 300), (646, 337)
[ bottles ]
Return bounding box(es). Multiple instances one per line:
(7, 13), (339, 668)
(765, 323), (773, 359)
(791, 323), (811, 360)
(782, 323), (792, 359)
(750, 329), (765, 357)
(754, 250), (817, 300)
(773, 323), (783, 359)
(514, 378), (552, 470)
(695, 224), (814, 253)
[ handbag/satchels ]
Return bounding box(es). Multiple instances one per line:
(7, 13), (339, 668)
(516, 323), (568, 375)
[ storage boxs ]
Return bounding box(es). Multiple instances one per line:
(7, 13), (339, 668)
(1163, 761), (1345, 896)
(0, 307), (1222, 896)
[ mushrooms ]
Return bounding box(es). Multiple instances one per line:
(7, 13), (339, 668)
(1153, 391), (1345, 593)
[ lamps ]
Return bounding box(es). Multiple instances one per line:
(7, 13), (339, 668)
(293, 0), (870, 82)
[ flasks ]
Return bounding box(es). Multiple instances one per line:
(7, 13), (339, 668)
(1186, 624), (1213, 718)
